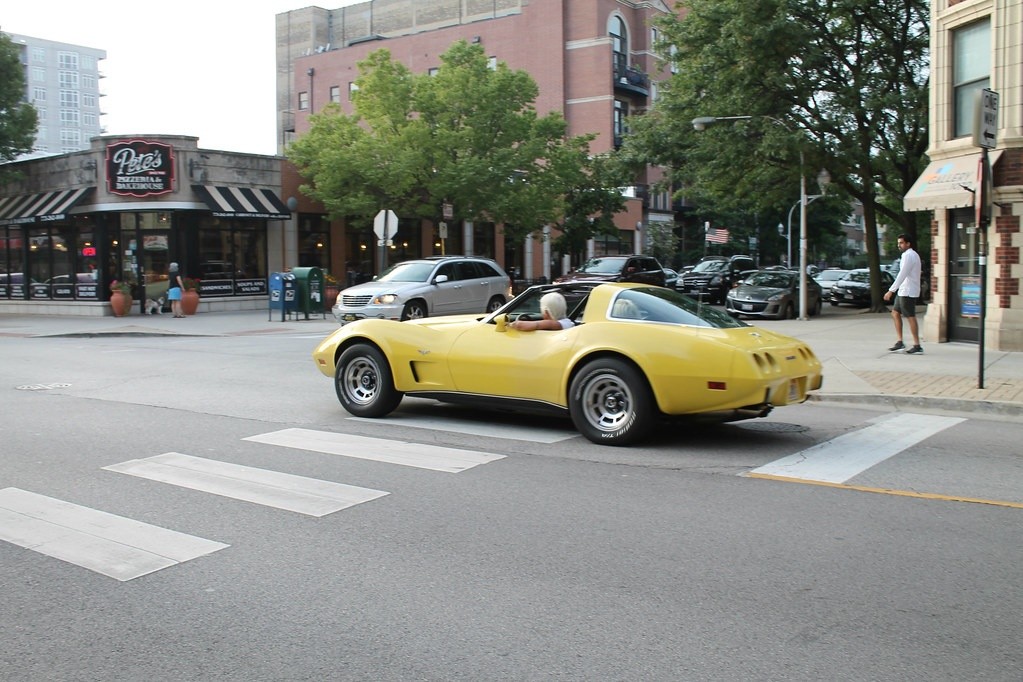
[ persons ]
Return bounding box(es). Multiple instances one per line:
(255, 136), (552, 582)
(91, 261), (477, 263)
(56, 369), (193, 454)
(883, 233), (923, 355)
(168, 262), (186, 318)
(615, 299), (641, 318)
(507, 292), (576, 332)
(344, 260), (366, 288)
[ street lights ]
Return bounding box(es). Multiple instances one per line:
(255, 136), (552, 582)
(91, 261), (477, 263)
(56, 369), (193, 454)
(689, 113), (809, 323)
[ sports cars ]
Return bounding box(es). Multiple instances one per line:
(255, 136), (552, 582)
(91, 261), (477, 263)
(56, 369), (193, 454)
(311, 280), (824, 447)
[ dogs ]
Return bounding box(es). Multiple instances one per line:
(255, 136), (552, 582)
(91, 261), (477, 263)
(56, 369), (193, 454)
(144, 297), (165, 315)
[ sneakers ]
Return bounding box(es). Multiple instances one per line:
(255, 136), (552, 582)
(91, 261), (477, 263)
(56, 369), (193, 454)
(889, 343), (905, 351)
(906, 346), (923, 354)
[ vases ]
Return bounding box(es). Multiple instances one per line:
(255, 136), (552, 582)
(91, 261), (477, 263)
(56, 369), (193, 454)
(111, 290), (132, 317)
(180, 287), (199, 314)
(324, 285), (339, 310)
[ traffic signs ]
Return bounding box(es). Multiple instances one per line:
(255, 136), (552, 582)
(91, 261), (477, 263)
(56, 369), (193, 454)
(970, 87), (1000, 151)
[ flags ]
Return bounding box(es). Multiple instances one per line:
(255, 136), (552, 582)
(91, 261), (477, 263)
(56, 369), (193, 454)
(705, 226), (729, 244)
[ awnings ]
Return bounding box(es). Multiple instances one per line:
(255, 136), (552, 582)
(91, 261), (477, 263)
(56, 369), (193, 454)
(0, 187), (97, 227)
(903, 149), (1005, 212)
(190, 184), (292, 221)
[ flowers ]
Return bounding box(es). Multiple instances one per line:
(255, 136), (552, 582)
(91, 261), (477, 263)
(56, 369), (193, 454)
(110, 280), (130, 314)
(323, 274), (339, 286)
(182, 277), (201, 293)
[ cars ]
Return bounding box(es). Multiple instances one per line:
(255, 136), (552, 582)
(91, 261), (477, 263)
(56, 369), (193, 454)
(663, 253), (930, 320)
(331, 252), (516, 328)
(0, 271), (96, 299)
(552, 253), (666, 293)
